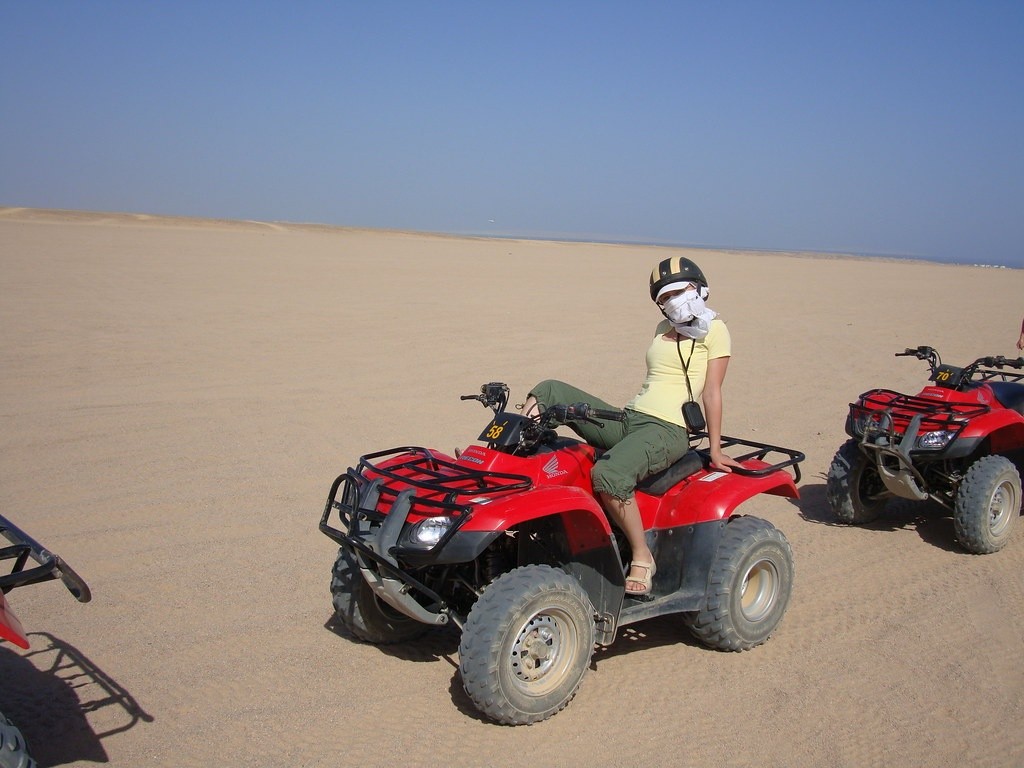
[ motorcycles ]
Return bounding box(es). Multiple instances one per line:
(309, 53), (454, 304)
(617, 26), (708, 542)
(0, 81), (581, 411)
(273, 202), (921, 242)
(316, 381), (806, 727)
(824, 344), (1024, 555)
(0, 514), (92, 768)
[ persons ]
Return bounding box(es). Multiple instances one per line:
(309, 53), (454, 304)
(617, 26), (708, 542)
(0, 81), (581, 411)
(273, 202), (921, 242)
(520, 256), (744, 594)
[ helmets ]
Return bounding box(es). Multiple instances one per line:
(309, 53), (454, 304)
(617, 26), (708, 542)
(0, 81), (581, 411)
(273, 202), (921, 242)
(649, 256), (709, 302)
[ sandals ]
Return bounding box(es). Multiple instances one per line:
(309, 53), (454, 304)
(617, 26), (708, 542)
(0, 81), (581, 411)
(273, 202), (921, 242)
(625, 547), (656, 594)
(455, 446), (464, 459)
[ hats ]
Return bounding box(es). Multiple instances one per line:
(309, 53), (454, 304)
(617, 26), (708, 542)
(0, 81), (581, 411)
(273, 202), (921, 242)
(656, 281), (698, 303)
